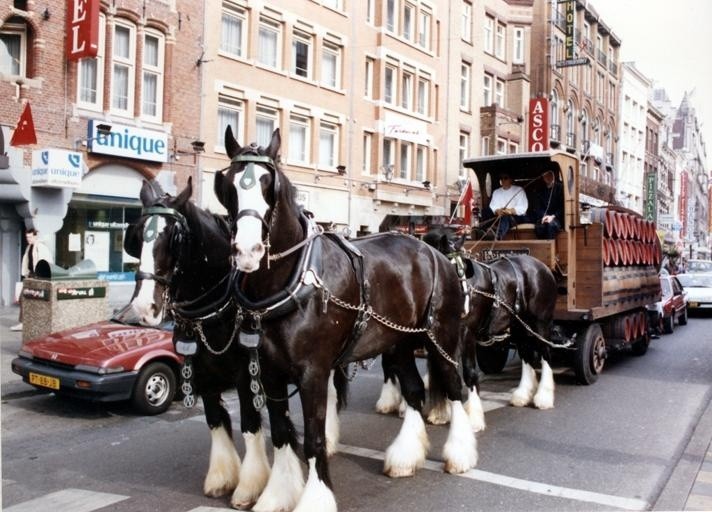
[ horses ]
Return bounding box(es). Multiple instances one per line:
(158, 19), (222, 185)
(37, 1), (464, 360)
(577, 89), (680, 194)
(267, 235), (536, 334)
(372, 221), (559, 433)
(214, 122), (481, 512)
(128, 173), (352, 510)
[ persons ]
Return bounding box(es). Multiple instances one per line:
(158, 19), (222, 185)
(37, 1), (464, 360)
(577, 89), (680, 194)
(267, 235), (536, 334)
(478, 173), (529, 240)
(532, 168), (564, 239)
(10, 228), (53, 331)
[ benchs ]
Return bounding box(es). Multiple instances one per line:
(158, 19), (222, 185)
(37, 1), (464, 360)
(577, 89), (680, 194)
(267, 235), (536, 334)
(486, 223), (567, 232)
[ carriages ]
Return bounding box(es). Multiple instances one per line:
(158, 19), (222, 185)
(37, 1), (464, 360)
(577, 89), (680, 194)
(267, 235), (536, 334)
(118, 123), (665, 511)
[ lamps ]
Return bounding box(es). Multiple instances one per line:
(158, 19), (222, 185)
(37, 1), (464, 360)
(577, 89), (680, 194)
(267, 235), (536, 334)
(499, 114), (525, 126)
(74, 125), (112, 153)
(401, 180), (431, 195)
(168, 141), (206, 162)
(314, 166), (347, 184)
(41, 8), (53, 22)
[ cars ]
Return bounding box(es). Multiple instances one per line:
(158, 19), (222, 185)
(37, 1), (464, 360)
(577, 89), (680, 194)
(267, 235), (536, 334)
(653, 258), (711, 334)
(11, 304), (198, 415)
